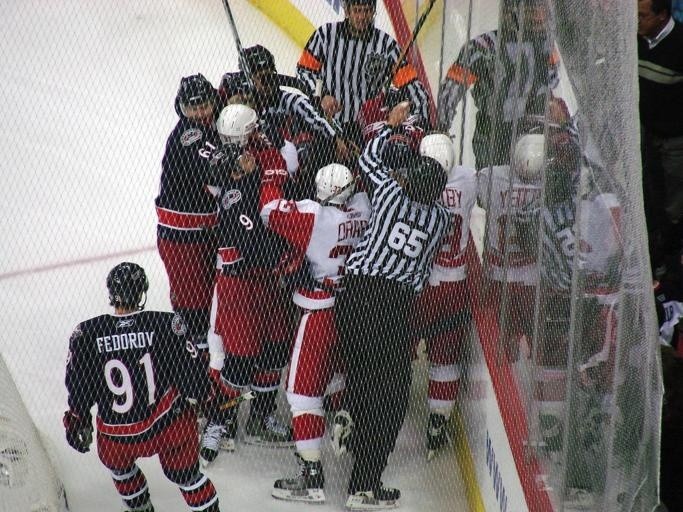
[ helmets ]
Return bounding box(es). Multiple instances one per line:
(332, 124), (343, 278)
(175, 73), (222, 123)
(237, 45), (276, 82)
(105, 262), (148, 307)
(509, 133), (546, 184)
(215, 103), (260, 149)
(313, 162), (355, 205)
(547, 135), (582, 169)
(546, 157), (575, 204)
(418, 133), (456, 174)
(401, 156), (448, 202)
(379, 131), (419, 172)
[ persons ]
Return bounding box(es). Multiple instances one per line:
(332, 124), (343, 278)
(436, 0), (633, 512)
(155, 1), (477, 511)
(63, 262), (220, 512)
(638, 0), (683, 512)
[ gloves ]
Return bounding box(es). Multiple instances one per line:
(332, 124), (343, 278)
(62, 410), (96, 454)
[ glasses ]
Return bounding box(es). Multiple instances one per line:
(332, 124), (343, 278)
(636, 12), (660, 24)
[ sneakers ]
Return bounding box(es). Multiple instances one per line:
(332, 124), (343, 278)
(220, 413), (240, 454)
(424, 412), (449, 462)
(270, 460), (328, 505)
(242, 405), (297, 450)
(344, 479), (403, 512)
(196, 417), (228, 471)
(330, 409), (355, 463)
